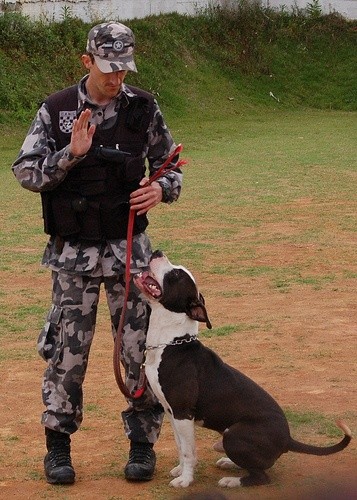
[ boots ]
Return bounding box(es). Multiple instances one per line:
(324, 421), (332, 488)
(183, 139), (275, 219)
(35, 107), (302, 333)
(42, 426), (77, 486)
(123, 430), (159, 482)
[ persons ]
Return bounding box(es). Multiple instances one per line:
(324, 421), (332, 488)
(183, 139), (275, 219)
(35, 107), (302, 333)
(11, 20), (184, 485)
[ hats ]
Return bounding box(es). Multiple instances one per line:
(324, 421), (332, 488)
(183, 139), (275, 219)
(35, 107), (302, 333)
(83, 19), (140, 75)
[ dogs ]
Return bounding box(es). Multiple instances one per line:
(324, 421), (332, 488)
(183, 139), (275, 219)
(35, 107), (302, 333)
(132, 249), (352, 488)
